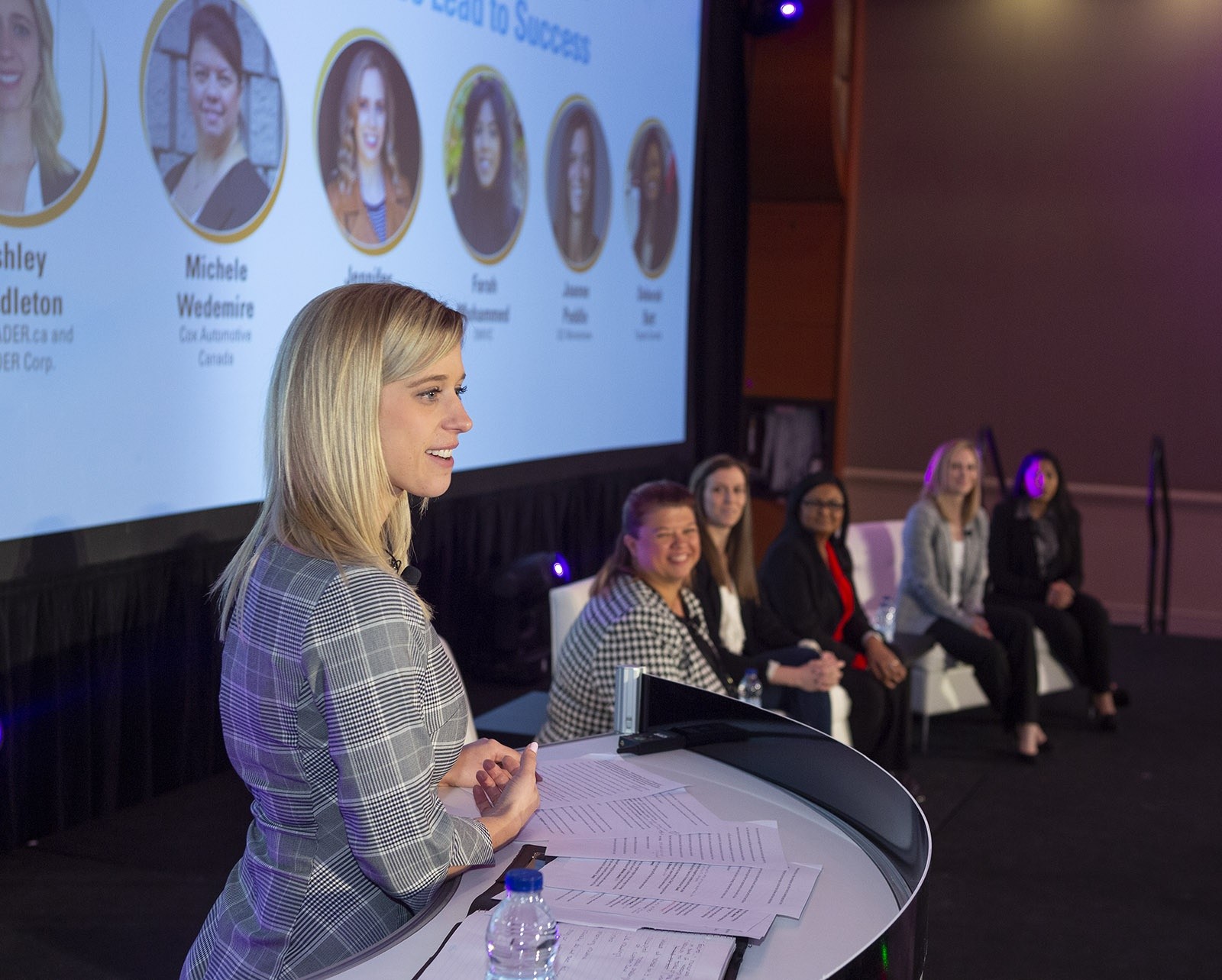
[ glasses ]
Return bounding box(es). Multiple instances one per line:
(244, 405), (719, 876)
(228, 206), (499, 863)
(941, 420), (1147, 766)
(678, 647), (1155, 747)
(800, 498), (844, 512)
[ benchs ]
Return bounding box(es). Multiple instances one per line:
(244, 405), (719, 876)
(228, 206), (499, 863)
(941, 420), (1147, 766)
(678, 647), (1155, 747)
(836, 519), (1078, 758)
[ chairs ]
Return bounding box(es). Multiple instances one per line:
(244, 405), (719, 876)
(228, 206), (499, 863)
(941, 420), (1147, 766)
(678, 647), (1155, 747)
(548, 573), (853, 747)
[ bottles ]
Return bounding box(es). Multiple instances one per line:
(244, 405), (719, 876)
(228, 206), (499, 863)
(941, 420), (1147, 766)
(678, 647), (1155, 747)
(738, 667), (763, 708)
(876, 594), (897, 643)
(484, 868), (559, 980)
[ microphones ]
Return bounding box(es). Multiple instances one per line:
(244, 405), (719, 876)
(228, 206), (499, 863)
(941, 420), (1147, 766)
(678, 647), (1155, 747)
(401, 565), (421, 587)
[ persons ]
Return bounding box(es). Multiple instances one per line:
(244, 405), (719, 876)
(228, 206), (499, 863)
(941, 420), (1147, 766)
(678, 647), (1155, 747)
(532, 438), (1133, 803)
(1, 0), (673, 269)
(177, 282), (542, 980)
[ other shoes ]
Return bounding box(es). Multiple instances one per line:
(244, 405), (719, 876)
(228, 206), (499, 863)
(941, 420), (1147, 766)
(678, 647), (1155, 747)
(1013, 728), (1050, 763)
(1095, 699), (1121, 735)
